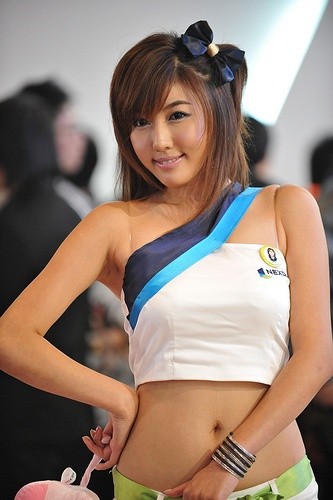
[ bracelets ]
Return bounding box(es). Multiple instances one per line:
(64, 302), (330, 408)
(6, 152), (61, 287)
(210, 431), (257, 480)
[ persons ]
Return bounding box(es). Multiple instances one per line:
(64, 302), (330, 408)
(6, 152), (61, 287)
(0, 19), (333, 500)
(0, 77), (333, 500)
